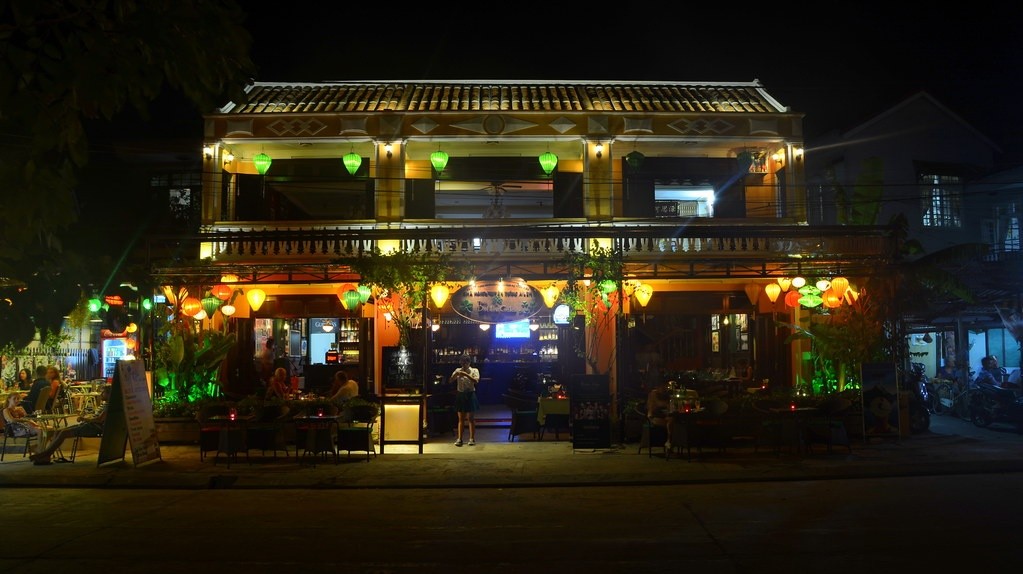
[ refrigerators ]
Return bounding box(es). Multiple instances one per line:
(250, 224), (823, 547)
(100, 329), (134, 384)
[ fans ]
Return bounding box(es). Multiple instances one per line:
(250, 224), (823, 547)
(478, 181), (522, 193)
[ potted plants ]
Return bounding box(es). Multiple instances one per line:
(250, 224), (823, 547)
(250, 395), (286, 420)
(342, 397), (372, 419)
(318, 396), (347, 416)
(291, 397), (320, 417)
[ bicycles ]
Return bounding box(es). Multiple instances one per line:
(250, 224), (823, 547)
(911, 368), (1023, 428)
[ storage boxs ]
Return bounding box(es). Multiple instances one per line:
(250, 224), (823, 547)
(343, 349), (360, 362)
(325, 349), (338, 363)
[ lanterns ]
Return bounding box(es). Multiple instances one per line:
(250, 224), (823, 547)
(253, 153), (272, 177)
(343, 153), (362, 175)
(596, 279), (654, 308)
(744, 277), (860, 309)
(540, 285), (559, 309)
(430, 285), (449, 309)
(336, 284), (371, 312)
(430, 150), (448, 174)
(182, 286), (265, 322)
(539, 151), (558, 178)
(625, 151), (644, 172)
(738, 152), (755, 173)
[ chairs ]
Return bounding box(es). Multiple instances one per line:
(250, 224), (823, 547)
(500, 388), (541, 442)
(1, 378), (106, 465)
(196, 393), (383, 472)
(638, 380), (854, 464)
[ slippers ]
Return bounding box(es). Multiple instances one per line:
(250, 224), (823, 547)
(53, 457), (73, 463)
(33, 460), (52, 465)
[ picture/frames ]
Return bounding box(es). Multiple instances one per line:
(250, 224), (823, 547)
(289, 330), (302, 358)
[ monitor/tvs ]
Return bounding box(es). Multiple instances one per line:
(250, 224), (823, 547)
(495, 318), (531, 339)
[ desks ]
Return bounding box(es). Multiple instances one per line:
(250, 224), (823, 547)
(98, 383), (112, 386)
(782, 407), (820, 457)
(71, 385), (98, 405)
(19, 414), (78, 458)
(208, 413), (257, 458)
(292, 410), (343, 466)
(74, 392), (101, 414)
(537, 396), (570, 441)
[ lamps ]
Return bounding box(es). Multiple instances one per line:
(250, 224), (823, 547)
(253, 143), (273, 175)
(737, 142), (755, 175)
(479, 322), (490, 331)
(336, 282), (396, 328)
(625, 142), (645, 172)
(224, 149), (234, 166)
(384, 141), (392, 158)
(489, 187), (505, 196)
(430, 141), (449, 175)
(540, 273), (654, 330)
(204, 142), (212, 161)
(87, 287), (154, 349)
(430, 282), (449, 311)
(322, 319), (333, 333)
(595, 139), (604, 159)
(342, 143), (362, 175)
(482, 199), (513, 219)
(181, 273), (267, 321)
(772, 148), (782, 165)
(538, 141), (559, 178)
(431, 318), (441, 332)
(795, 144), (802, 161)
(745, 272), (860, 314)
(528, 317), (539, 332)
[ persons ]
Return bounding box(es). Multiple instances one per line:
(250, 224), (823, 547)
(265, 368), (288, 401)
(741, 358), (753, 387)
(328, 371), (360, 404)
(448, 356), (481, 446)
(977, 355), (1006, 389)
(647, 381), (674, 453)
(2, 366), (112, 464)
(937, 358), (958, 379)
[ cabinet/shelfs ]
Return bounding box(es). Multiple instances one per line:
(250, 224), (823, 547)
(335, 318), (360, 353)
(539, 309), (563, 363)
(428, 313), (497, 364)
(496, 338), (540, 363)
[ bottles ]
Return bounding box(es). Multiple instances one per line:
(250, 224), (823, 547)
(669, 386), (688, 413)
(338, 319), (361, 366)
(104, 345), (125, 380)
(430, 344), (537, 363)
(538, 322), (559, 362)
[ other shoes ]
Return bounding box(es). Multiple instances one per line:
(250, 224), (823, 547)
(468, 439), (476, 444)
(455, 439), (463, 446)
